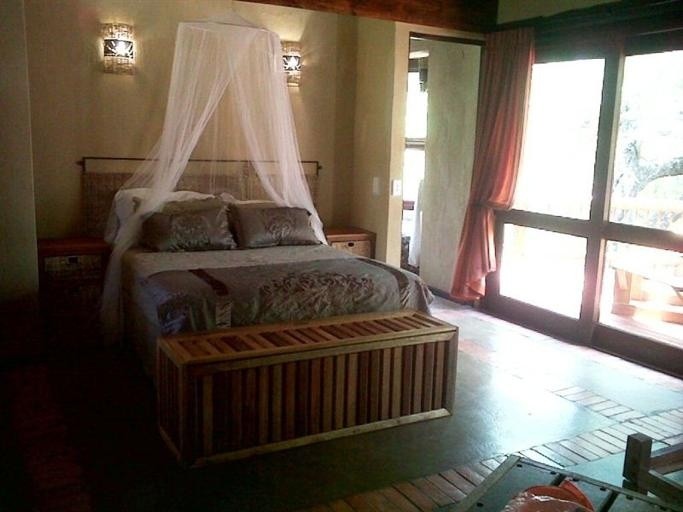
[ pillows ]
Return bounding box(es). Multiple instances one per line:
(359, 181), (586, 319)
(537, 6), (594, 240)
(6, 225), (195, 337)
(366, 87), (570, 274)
(115, 189), (322, 252)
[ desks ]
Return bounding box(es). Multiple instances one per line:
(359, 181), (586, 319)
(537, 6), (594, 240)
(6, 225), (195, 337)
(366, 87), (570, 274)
(450, 454), (683, 512)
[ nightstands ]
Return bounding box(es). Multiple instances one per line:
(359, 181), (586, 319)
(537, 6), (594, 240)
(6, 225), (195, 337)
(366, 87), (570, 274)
(324, 226), (376, 260)
(37, 238), (107, 312)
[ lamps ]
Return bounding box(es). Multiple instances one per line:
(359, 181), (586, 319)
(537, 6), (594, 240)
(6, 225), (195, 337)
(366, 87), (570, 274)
(96, 22), (139, 76)
(280, 40), (304, 88)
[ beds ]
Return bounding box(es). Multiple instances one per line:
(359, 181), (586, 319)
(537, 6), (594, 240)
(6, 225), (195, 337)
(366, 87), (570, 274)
(80, 156), (434, 388)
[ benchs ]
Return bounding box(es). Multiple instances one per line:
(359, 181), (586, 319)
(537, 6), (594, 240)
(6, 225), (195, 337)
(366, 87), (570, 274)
(157, 308), (460, 476)
